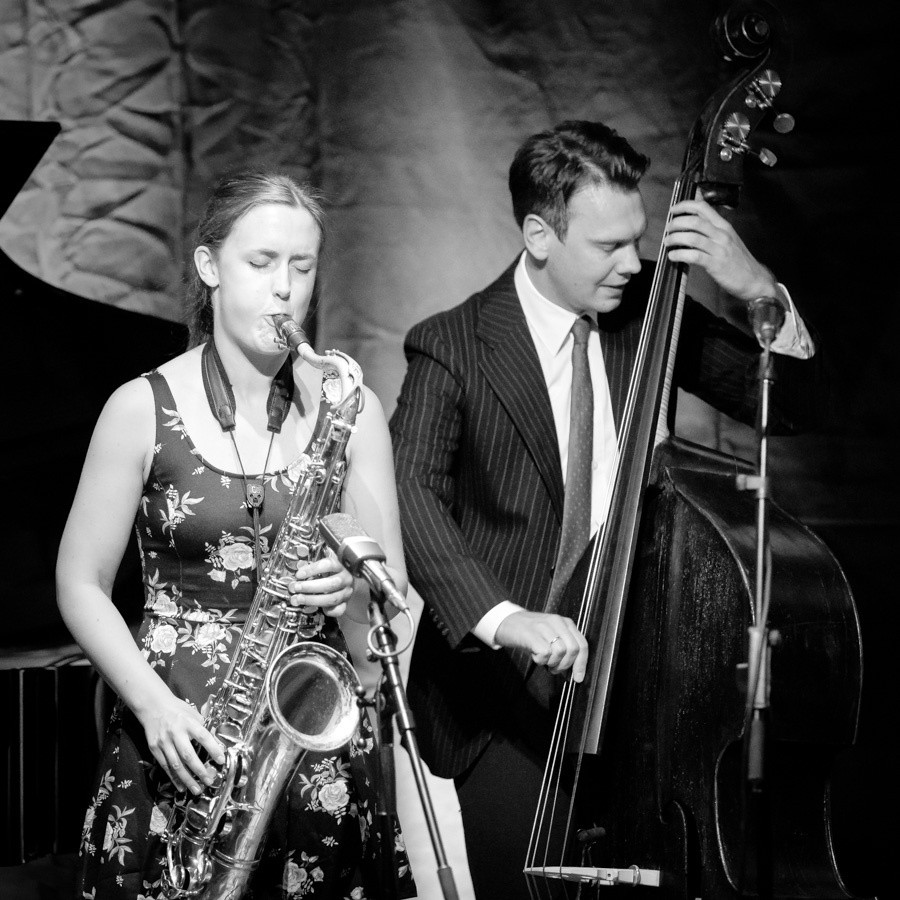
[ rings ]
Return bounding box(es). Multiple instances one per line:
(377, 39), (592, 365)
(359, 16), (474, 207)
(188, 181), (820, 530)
(550, 635), (560, 645)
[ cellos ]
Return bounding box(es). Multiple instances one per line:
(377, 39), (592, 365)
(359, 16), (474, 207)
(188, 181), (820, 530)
(519, 10), (867, 900)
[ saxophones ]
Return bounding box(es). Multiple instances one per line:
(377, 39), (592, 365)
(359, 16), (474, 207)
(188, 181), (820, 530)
(159, 313), (367, 900)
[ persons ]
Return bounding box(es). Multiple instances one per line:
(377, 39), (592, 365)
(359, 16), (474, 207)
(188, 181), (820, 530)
(389, 117), (833, 900)
(56, 167), (422, 900)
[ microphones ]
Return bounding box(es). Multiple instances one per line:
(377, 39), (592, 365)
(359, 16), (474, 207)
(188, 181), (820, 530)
(317, 513), (412, 612)
(749, 296), (786, 344)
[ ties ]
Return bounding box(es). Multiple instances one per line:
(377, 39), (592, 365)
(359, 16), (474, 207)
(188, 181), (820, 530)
(547, 319), (593, 622)
